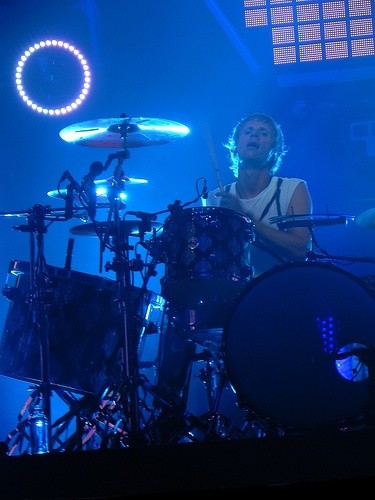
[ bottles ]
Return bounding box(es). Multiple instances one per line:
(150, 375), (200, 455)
(30, 405), (49, 454)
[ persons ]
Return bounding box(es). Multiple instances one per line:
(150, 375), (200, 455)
(149, 112), (313, 442)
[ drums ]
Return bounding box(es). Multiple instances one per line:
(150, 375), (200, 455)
(0, 258), (166, 399)
(221, 261), (375, 433)
(159, 207), (255, 327)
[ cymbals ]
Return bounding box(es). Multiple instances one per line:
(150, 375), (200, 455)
(268, 214), (355, 222)
(356, 208), (375, 229)
(46, 178), (148, 203)
(70, 220), (165, 238)
(58, 116), (190, 148)
